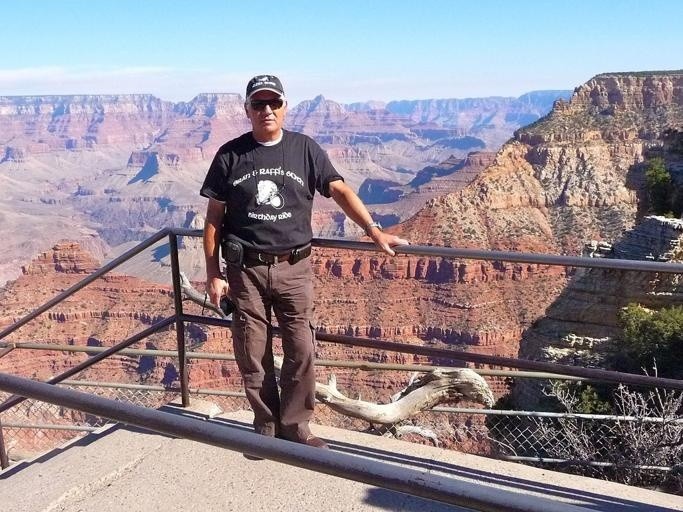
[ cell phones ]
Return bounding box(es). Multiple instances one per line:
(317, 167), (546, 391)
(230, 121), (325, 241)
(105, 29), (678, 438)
(219, 295), (235, 317)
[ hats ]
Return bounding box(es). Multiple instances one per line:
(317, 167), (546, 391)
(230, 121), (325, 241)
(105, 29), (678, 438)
(246, 75), (284, 99)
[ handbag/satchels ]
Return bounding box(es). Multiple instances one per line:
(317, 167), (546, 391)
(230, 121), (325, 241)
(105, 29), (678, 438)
(222, 239), (243, 267)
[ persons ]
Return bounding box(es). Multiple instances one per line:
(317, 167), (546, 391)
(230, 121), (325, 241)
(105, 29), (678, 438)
(199, 74), (410, 460)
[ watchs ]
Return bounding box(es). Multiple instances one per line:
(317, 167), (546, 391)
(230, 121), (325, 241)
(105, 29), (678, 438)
(363, 221), (383, 238)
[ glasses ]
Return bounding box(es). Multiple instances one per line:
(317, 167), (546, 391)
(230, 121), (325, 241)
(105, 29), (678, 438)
(250, 100), (282, 110)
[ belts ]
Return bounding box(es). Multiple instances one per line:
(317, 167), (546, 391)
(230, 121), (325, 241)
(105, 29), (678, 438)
(247, 251), (291, 264)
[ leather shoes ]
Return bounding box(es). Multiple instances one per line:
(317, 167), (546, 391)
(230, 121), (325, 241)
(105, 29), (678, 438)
(295, 434), (329, 448)
